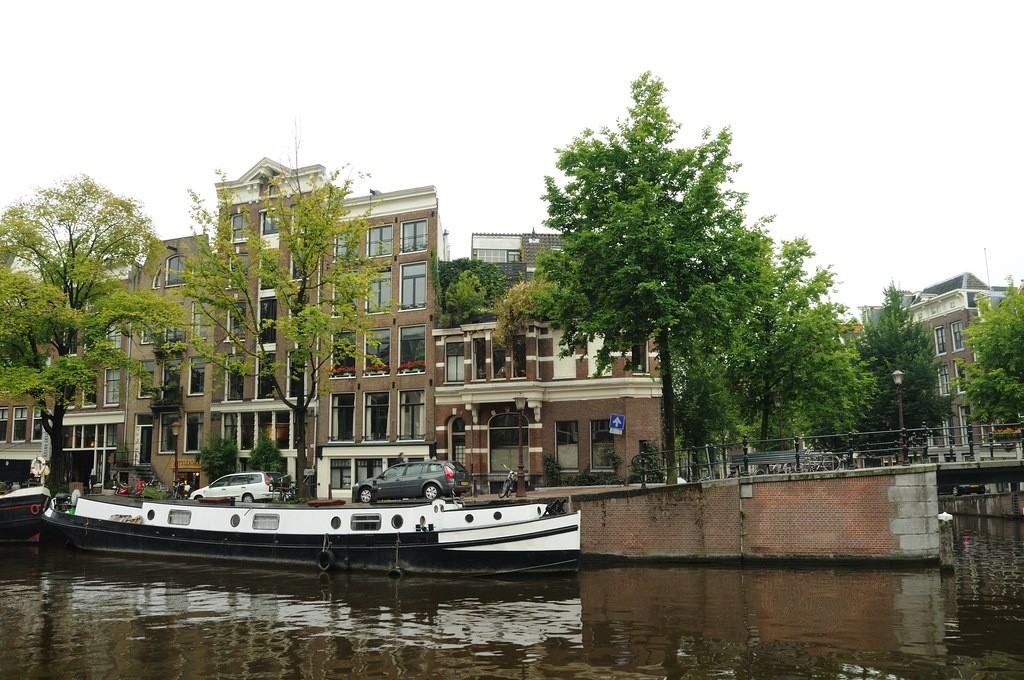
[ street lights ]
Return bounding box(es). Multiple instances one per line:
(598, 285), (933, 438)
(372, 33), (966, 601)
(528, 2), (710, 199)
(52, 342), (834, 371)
(169, 419), (184, 485)
(772, 391), (785, 450)
(891, 368), (906, 465)
(512, 392), (530, 497)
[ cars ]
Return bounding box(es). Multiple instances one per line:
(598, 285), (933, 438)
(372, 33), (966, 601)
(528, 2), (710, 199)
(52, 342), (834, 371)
(937, 482), (986, 496)
(188, 471), (291, 503)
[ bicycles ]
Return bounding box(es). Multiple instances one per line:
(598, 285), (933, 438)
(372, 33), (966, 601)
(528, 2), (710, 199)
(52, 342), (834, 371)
(273, 473), (297, 504)
(726, 442), (842, 478)
(498, 464), (518, 498)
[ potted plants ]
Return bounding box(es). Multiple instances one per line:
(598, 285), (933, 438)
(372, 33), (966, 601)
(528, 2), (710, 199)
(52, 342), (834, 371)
(115, 453), (127, 467)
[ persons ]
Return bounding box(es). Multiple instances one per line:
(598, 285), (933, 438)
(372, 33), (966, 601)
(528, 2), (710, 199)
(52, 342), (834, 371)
(399, 453), (409, 463)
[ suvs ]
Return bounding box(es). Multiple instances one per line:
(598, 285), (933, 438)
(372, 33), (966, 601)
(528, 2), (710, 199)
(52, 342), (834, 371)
(353, 459), (472, 504)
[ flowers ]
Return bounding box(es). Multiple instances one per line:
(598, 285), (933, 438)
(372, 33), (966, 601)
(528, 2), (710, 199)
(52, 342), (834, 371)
(334, 361), (427, 373)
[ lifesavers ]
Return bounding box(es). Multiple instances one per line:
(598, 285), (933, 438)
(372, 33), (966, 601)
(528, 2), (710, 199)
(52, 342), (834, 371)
(317, 549), (336, 572)
(30, 457), (46, 476)
(386, 568), (406, 578)
(30, 504), (40, 515)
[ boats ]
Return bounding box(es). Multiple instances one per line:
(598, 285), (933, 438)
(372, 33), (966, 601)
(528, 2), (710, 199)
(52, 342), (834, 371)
(0, 480), (54, 544)
(42, 483), (582, 580)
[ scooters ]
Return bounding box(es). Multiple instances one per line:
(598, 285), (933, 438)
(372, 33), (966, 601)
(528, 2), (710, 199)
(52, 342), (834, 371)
(112, 477), (191, 500)
